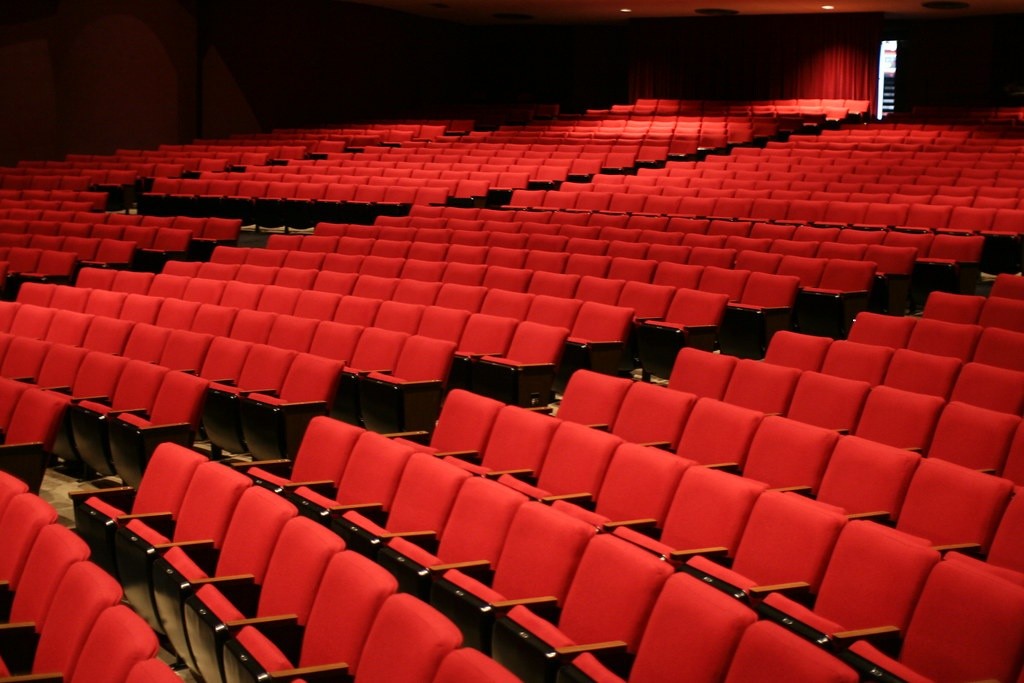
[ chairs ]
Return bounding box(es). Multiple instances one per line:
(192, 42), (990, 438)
(0, 97), (1024, 683)
